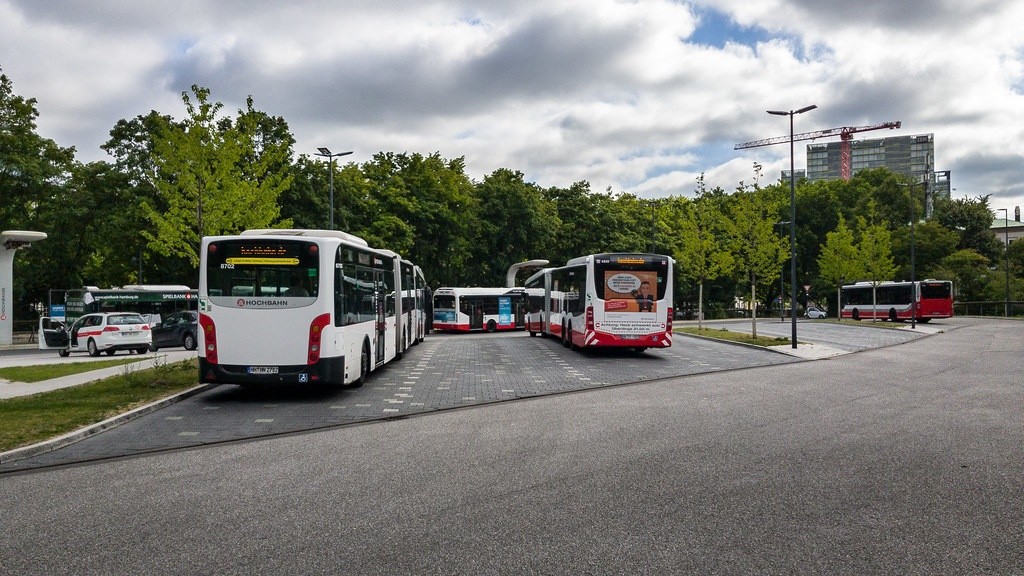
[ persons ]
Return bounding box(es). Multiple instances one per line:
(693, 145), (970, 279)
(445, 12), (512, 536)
(283, 276), (309, 297)
(85, 318), (95, 326)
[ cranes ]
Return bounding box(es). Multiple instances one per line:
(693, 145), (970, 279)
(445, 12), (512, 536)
(734, 121), (902, 182)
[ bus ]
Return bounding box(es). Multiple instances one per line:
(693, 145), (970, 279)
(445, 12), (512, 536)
(197, 229), (433, 389)
(344, 276), (388, 323)
(521, 253), (673, 350)
(386, 289), (425, 316)
(432, 287), (525, 333)
(64, 284), (289, 328)
(840, 279), (954, 324)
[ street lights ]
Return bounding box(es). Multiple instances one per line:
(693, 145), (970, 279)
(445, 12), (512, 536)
(896, 181), (926, 329)
(766, 105), (818, 349)
(998, 209), (1010, 317)
(314, 148), (353, 229)
(779, 222), (791, 321)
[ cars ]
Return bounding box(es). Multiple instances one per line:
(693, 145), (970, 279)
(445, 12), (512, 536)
(147, 311), (199, 351)
(803, 307), (827, 319)
(38, 312), (152, 357)
